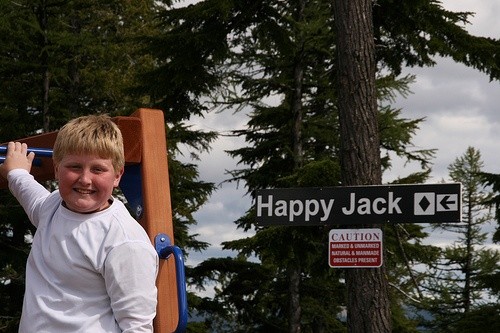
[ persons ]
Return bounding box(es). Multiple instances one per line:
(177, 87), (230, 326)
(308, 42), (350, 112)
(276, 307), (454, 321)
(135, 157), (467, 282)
(0, 114), (160, 333)
